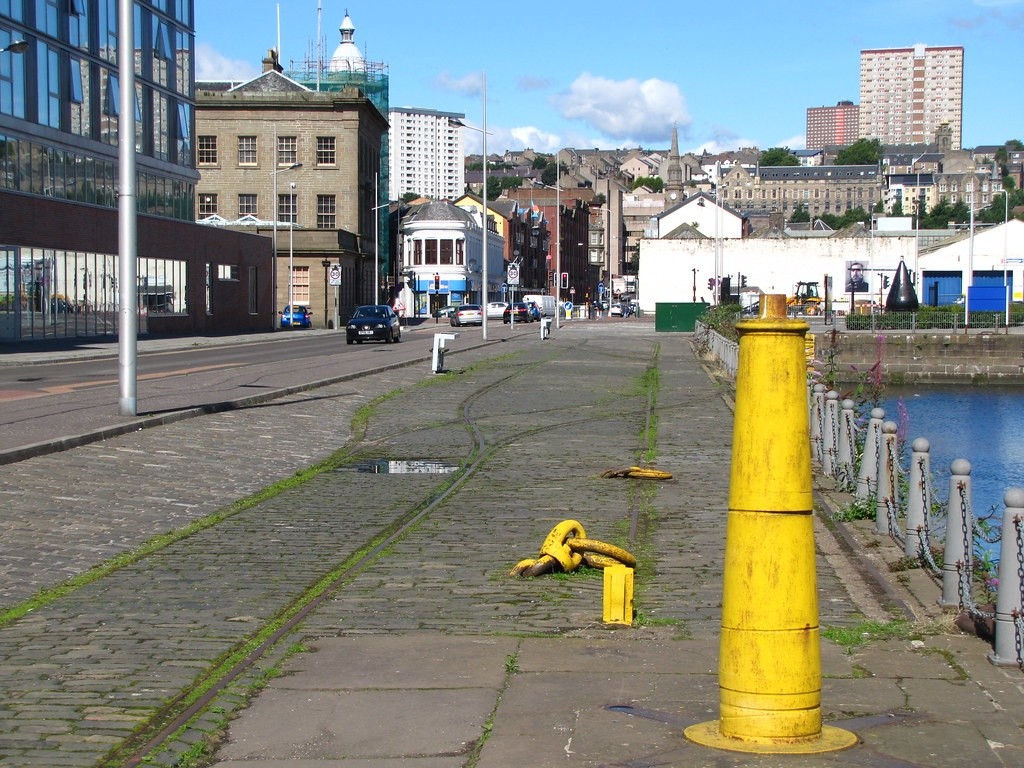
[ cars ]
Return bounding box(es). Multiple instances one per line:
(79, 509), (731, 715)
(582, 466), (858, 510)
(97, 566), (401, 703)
(434, 303), (540, 326)
(278, 305), (313, 328)
(345, 305), (401, 344)
(596, 296), (638, 316)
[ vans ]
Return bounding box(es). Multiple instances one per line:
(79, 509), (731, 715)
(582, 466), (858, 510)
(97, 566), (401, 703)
(524, 294), (561, 317)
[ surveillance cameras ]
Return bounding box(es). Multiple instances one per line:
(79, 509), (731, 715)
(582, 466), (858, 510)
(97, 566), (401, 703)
(291, 183), (296, 189)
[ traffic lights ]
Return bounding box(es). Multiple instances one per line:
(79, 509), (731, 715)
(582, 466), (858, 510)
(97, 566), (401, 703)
(708, 278), (713, 290)
(884, 275), (890, 289)
(435, 276), (440, 289)
(741, 275), (748, 289)
(561, 273), (569, 289)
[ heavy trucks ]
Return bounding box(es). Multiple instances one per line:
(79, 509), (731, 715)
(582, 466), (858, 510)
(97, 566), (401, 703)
(613, 275), (638, 296)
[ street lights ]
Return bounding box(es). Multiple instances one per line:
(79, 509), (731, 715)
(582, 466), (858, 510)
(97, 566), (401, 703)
(289, 182), (296, 326)
(996, 189), (1007, 286)
(372, 201), (398, 311)
(273, 162), (303, 331)
(870, 201), (876, 313)
(449, 118), (492, 340)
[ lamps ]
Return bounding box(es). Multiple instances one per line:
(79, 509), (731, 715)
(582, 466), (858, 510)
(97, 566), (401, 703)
(697, 198), (705, 208)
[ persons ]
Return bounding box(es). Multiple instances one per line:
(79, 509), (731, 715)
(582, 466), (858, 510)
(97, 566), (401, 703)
(845, 263), (868, 292)
(622, 304), (636, 317)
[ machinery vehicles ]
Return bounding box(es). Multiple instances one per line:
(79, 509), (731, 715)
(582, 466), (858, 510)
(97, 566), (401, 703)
(784, 281), (820, 316)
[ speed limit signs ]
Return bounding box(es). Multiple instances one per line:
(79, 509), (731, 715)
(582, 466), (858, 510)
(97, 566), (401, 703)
(509, 269), (518, 278)
(331, 269), (340, 280)
